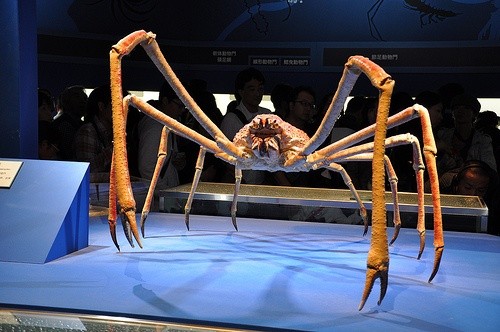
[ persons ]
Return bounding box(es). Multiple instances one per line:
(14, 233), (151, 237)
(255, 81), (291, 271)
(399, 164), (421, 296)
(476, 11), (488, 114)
(72, 84), (112, 183)
(38, 64), (500, 235)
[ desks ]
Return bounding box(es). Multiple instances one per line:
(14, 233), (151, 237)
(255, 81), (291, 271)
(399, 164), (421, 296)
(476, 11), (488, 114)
(159, 182), (488, 233)
(0, 205), (500, 332)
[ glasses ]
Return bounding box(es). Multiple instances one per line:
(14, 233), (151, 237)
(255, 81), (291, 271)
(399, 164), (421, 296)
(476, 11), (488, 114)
(244, 85), (264, 94)
(170, 101), (184, 108)
(296, 100), (319, 110)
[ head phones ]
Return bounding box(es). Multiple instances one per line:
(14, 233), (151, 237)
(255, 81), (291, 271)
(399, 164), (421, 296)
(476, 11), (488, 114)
(452, 160), (491, 186)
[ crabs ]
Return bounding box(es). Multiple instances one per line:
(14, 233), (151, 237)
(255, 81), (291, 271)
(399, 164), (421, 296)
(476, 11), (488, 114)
(108, 30), (445, 311)
